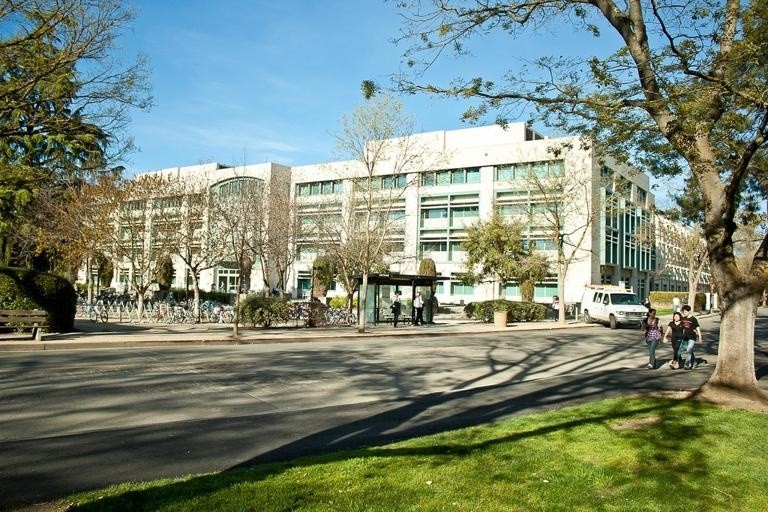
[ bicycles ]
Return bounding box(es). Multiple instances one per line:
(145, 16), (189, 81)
(71, 295), (357, 328)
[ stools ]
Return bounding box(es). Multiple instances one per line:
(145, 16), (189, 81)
(386, 318), (393, 326)
(404, 318), (411, 325)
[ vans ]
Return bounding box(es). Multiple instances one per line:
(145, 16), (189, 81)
(579, 284), (650, 332)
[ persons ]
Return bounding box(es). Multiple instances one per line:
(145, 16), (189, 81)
(663, 313), (684, 369)
(392, 292), (401, 327)
(413, 292), (427, 325)
(642, 309), (663, 369)
(644, 297), (650, 309)
(682, 298), (688, 305)
(552, 297), (559, 321)
(673, 295), (680, 312)
(680, 305), (702, 370)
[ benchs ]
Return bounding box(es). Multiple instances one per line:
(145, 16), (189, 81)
(0, 309), (50, 340)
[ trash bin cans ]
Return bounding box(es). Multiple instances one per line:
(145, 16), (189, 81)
(493, 310), (508, 328)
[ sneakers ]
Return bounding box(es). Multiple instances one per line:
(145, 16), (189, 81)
(669, 360), (697, 370)
(648, 363), (653, 369)
